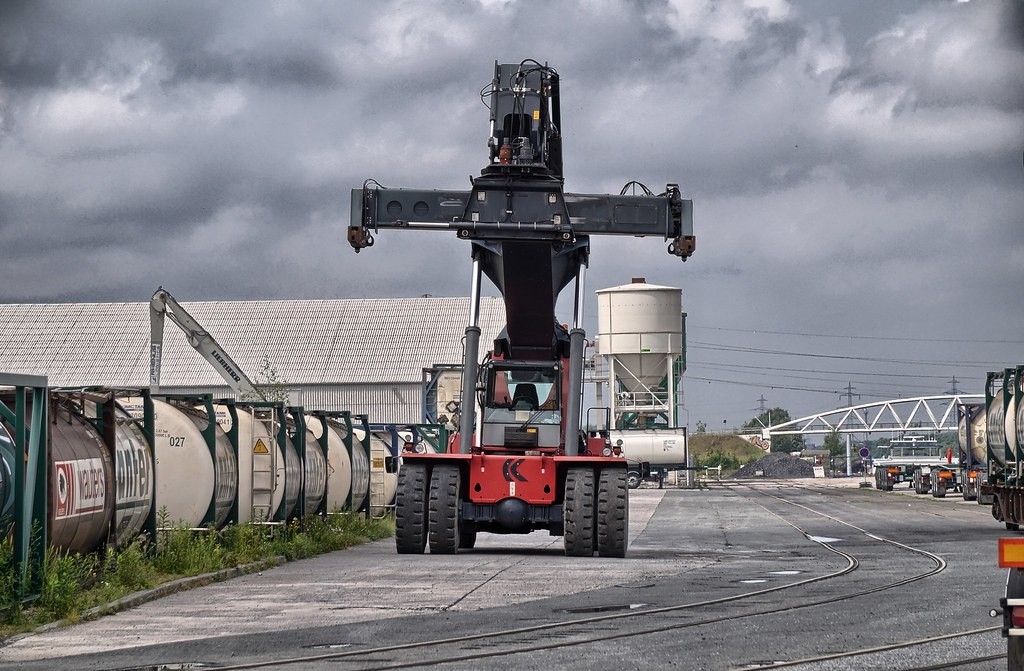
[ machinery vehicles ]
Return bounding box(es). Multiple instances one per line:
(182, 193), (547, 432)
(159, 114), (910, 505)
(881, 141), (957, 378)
(347, 56), (695, 559)
(149, 285), (272, 414)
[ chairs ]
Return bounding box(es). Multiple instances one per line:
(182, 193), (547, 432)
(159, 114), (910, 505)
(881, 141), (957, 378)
(502, 113), (533, 138)
(511, 383), (540, 414)
